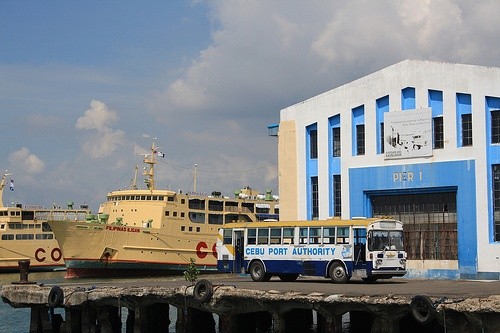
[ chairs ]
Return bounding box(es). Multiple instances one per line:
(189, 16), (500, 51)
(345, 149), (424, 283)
(372, 233), (389, 250)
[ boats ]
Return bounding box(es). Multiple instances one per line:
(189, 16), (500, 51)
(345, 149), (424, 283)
(0, 171), (92, 271)
(46, 142), (280, 279)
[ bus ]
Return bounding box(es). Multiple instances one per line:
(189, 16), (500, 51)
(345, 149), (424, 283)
(216, 217), (407, 283)
